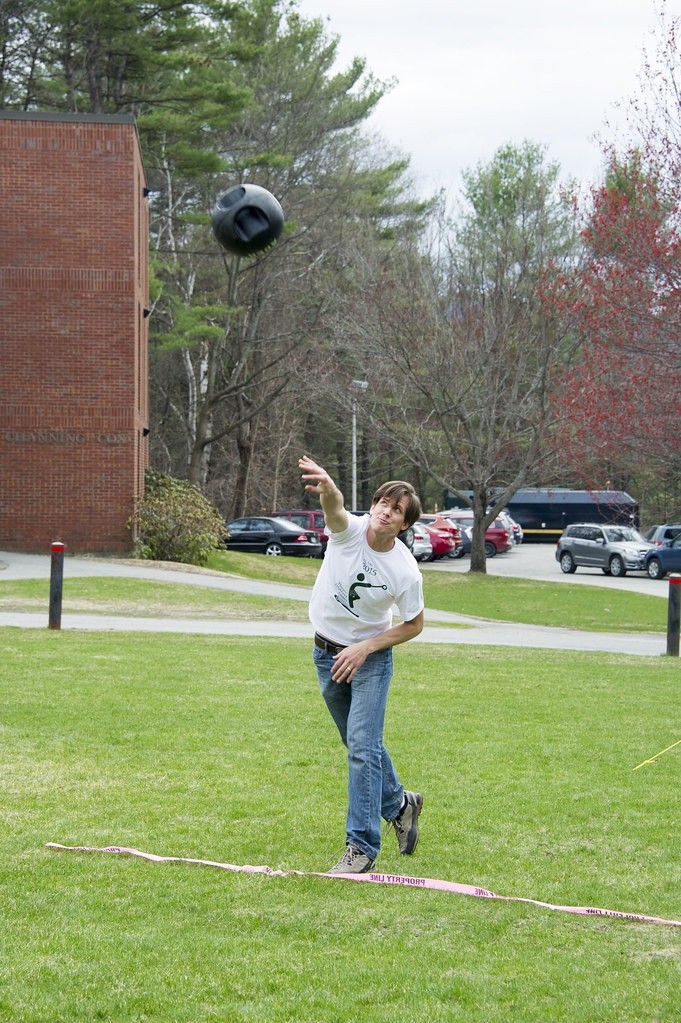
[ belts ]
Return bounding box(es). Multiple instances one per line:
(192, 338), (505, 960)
(314, 634), (344, 654)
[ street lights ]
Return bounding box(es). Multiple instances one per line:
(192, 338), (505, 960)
(349, 380), (369, 511)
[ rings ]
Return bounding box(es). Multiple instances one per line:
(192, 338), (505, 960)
(347, 667), (351, 672)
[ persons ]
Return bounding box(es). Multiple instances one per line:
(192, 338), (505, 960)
(298, 456), (426, 876)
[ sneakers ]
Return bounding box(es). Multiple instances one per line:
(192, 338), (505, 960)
(390, 790), (423, 855)
(327, 844), (376, 874)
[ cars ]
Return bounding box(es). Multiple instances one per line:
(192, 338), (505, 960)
(415, 521), (455, 563)
(435, 506), (523, 560)
(418, 514), (463, 560)
(411, 522), (432, 563)
(644, 533), (681, 580)
(447, 521), (474, 559)
(220, 516), (323, 557)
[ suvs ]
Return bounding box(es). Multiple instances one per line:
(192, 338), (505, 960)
(268, 509), (329, 559)
(555, 521), (655, 577)
(643, 523), (681, 548)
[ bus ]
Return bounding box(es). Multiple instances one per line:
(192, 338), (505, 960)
(349, 510), (415, 552)
